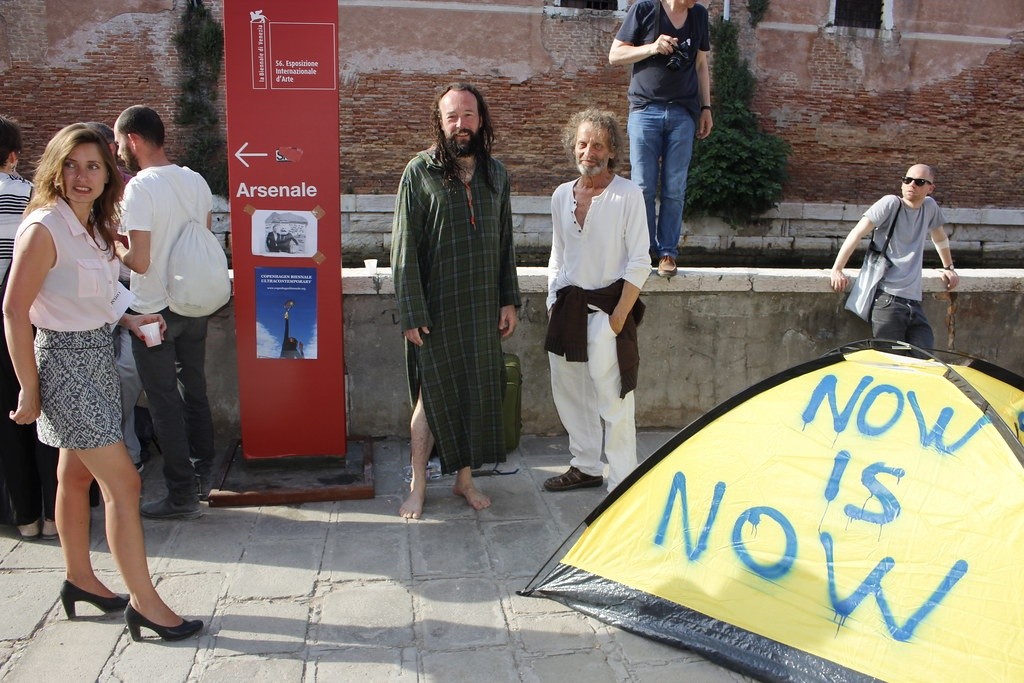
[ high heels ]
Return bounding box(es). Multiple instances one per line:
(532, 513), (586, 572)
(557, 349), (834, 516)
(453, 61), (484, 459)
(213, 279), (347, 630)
(123, 601), (203, 642)
(60, 580), (130, 619)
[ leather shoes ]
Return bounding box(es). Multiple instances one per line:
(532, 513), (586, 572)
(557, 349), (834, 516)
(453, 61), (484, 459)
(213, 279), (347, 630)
(140, 484), (202, 521)
(544, 466), (603, 491)
(198, 492), (209, 501)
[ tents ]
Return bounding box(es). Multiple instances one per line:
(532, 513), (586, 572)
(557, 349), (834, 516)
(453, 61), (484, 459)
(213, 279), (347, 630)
(516, 338), (1024, 683)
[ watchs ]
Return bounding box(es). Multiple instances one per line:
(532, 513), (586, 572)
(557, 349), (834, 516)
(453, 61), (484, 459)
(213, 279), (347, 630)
(944, 264), (955, 270)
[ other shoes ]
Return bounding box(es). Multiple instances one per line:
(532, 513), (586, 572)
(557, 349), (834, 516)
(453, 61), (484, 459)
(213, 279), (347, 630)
(658, 255), (677, 277)
(42, 517), (59, 539)
(16, 519), (41, 541)
(134, 463), (144, 472)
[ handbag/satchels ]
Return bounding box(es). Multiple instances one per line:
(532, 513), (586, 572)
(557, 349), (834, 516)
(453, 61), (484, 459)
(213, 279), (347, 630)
(844, 195), (902, 323)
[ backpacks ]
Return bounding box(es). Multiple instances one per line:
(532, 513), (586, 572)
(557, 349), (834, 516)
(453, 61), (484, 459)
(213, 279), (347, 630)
(138, 167), (231, 317)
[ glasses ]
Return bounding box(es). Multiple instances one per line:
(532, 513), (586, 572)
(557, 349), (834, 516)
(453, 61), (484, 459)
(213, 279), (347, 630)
(900, 176), (933, 187)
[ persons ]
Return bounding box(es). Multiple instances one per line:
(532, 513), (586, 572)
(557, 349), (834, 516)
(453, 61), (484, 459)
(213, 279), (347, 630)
(280, 312), (304, 358)
(389, 82), (522, 520)
(87, 105), (216, 522)
(608, 0), (713, 276)
(3, 122), (205, 643)
(545, 109), (652, 494)
(830, 164), (959, 359)
(0, 112), (98, 542)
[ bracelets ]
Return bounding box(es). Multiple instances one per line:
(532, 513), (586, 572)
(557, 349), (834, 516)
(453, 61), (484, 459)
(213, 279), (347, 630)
(701, 106), (710, 112)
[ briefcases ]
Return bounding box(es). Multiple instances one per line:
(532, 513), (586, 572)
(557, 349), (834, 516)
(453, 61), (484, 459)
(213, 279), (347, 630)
(496, 350), (521, 450)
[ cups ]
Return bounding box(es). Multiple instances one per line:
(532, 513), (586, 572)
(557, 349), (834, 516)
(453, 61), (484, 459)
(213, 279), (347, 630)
(138, 321), (161, 348)
(364, 258), (378, 274)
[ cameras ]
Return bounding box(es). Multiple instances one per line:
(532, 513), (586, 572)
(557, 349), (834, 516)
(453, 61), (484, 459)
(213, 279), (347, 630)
(664, 38), (691, 75)
